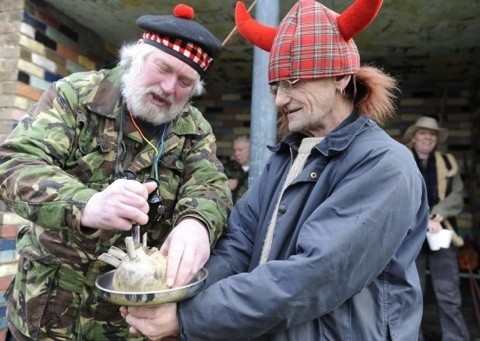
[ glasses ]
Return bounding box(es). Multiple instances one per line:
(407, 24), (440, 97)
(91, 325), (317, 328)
(269, 79), (301, 95)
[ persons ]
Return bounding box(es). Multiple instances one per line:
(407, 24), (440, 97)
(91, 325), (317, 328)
(0, 0), (233, 341)
(118, 0), (429, 341)
(224, 134), (251, 206)
(397, 118), (470, 341)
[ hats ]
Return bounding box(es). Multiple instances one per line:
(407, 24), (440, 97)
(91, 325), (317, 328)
(404, 116), (448, 146)
(234, 0), (382, 85)
(136, 3), (222, 82)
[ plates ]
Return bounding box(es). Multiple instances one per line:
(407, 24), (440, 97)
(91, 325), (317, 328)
(95, 266), (209, 307)
